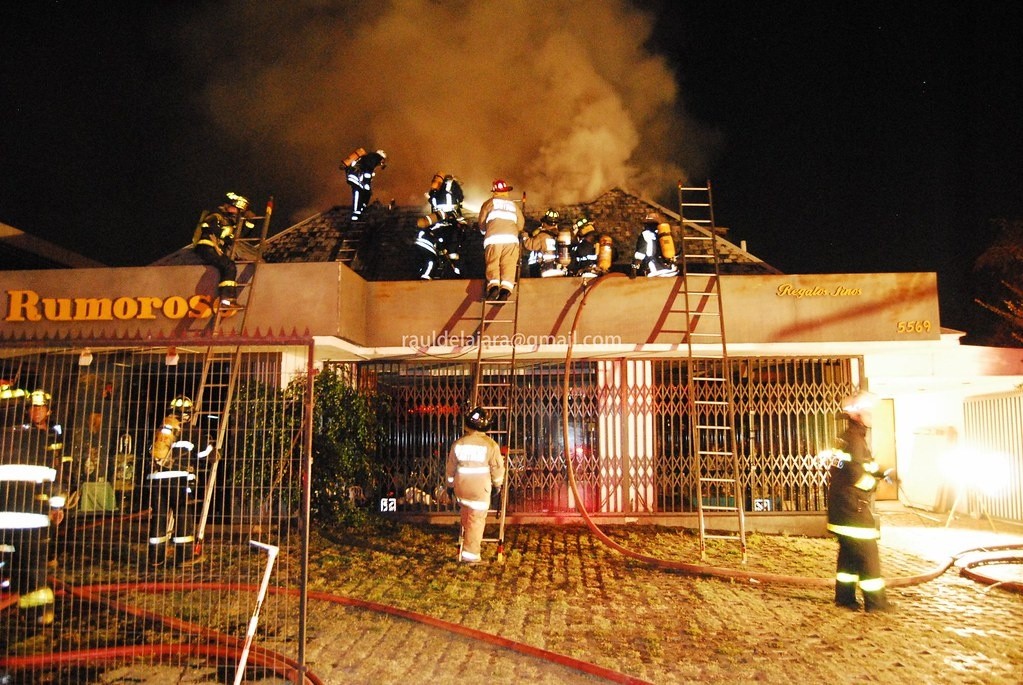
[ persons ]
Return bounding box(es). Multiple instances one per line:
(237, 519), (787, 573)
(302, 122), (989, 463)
(414, 169), (679, 300)
(443, 407), (505, 566)
(347, 147), (388, 224)
(825, 406), (901, 612)
(0, 389), (224, 639)
(194, 192), (256, 309)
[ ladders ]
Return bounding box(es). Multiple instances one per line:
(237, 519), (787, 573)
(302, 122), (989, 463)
(677, 180), (747, 565)
(458, 190), (529, 562)
(160, 196), (275, 558)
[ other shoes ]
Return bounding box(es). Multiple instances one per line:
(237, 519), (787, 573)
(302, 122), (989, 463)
(836, 599), (863, 611)
(495, 288), (510, 306)
(421, 274), (431, 280)
(175, 551), (207, 568)
(865, 601), (897, 613)
(220, 299), (243, 310)
(462, 556), (482, 563)
(351, 214), (366, 222)
(150, 551), (167, 566)
(486, 286), (499, 301)
(37, 607), (54, 625)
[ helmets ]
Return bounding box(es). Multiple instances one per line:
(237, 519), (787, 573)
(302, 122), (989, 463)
(490, 180), (513, 192)
(169, 394), (193, 412)
(375, 149), (387, 159)
(542, 208), (560, 224)
(224, 191), (250, 212)
(834, 390), (881, 419)
(465, 407), (491, 432)
(575, 217), (588, 230)
(26, 388), (52, 406)
